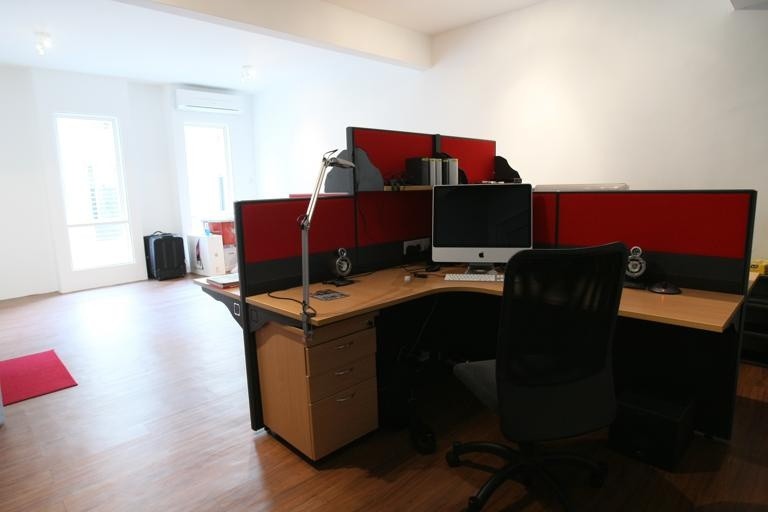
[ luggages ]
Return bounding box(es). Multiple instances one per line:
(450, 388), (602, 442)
(144, 231), (187, 281)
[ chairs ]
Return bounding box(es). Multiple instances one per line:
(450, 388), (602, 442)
(446, 240), (678, 512)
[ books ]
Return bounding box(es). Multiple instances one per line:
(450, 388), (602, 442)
(421, 153), (458, 186)
(206, 274), (239, 289)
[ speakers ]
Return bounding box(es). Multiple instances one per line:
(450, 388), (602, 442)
(622, 245), (651, 290)
(605, 379), (698, 471)
(321, 247), (354, 287)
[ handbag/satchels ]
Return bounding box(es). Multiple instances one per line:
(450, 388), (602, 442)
(188, 235), (225, 277)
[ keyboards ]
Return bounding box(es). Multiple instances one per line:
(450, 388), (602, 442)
(443, 273), (504, 282)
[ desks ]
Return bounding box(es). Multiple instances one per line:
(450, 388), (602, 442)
(193, 127), (768, 467)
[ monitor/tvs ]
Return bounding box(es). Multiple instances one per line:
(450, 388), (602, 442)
(431, 183), (534, 275)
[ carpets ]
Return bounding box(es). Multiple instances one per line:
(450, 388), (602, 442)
(0, 348), (79, 407)
